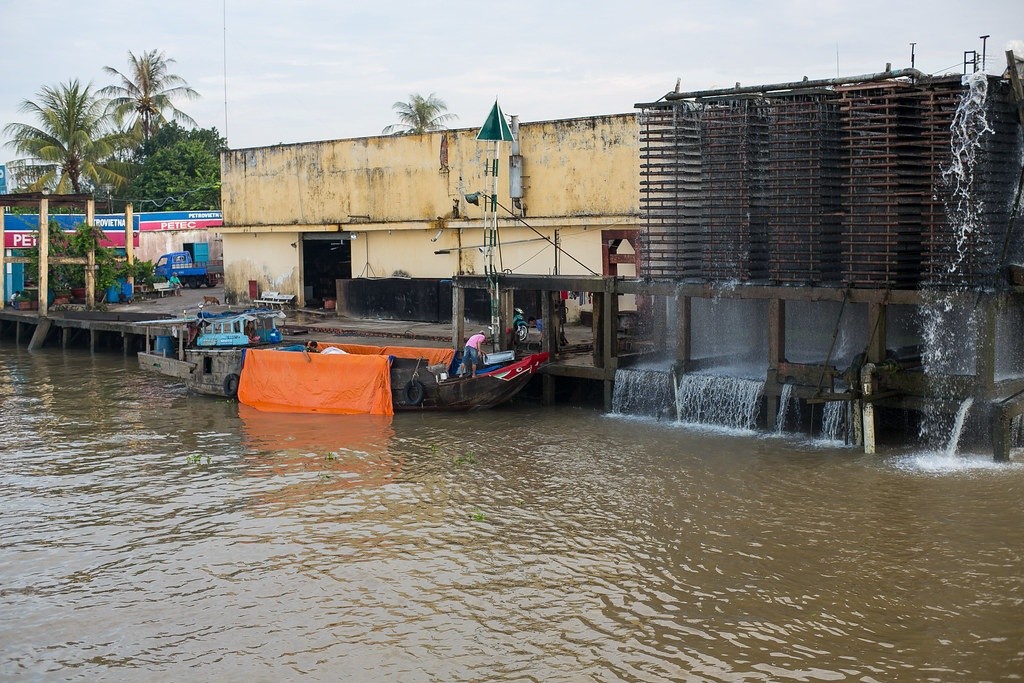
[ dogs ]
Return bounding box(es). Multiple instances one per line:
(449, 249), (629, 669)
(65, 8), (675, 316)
(204, 296), (220, 305)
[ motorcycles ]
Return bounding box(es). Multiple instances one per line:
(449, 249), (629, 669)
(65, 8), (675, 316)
(487, 305), (529, 341)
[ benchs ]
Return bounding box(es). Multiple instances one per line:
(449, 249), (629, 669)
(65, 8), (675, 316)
(153, 283), (177, 298)
(253, 291), (280, 309)
(266, 294), (296, 310)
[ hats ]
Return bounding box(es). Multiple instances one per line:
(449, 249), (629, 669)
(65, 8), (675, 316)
(478, 330), (485, 335)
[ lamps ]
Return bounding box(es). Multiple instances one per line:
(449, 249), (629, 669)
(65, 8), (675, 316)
(291, 237), (302, 248)
(350, 232), (360, 240)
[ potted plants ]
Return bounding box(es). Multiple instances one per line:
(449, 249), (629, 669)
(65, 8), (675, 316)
(133, 256), (159, 293)
(48, 275), (71, 304)
(63, 264), (86, 302)
(32, 294), (38, 310)
(14, 290), (32, 311)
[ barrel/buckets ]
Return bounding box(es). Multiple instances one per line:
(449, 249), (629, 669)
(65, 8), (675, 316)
(157, 336), (173, 356)
(107, 286), (120, 302)
(121, 283), (132, 302)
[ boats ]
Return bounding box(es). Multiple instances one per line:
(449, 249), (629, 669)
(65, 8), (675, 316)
(132, 309), (549, 408)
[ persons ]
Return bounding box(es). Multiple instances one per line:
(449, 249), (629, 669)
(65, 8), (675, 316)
(459, 330), (486, 378)
(303, 341), (320, 362)
(11, 291), (21, 306)
(169, 272), (183, 296)
(244, 321), (260, 343)
(528, 316), (542, 340)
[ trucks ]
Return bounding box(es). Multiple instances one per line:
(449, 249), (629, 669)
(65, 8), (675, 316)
(151, 251), (224, 288)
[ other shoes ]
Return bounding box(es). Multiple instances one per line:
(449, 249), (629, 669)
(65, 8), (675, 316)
(472, 375), (477, 379)
(459, 375), (463, 378)
